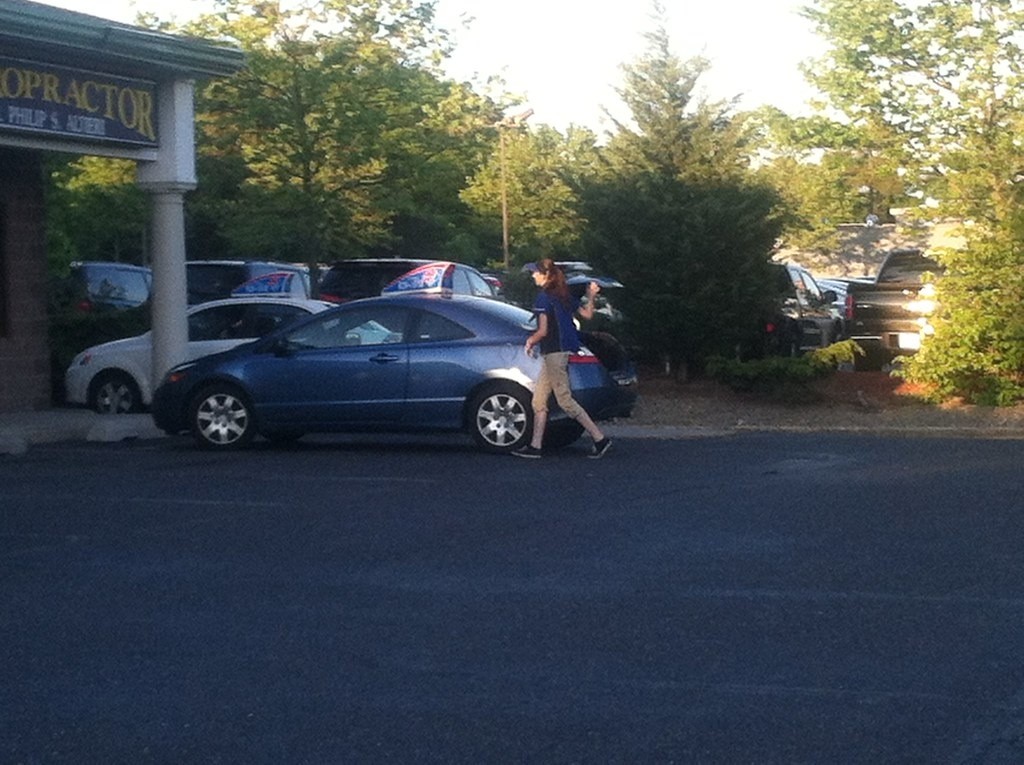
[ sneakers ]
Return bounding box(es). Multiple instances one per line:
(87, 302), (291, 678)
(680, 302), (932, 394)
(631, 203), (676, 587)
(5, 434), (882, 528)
(587, 437), (613, 458)
(510, 445), (543, 458)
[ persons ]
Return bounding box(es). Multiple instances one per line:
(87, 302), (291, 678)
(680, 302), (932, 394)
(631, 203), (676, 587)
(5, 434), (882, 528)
(510, 259), (612, 459)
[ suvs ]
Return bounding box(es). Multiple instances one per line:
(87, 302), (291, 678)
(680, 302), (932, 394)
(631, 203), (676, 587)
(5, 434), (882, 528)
(757, 242), (944, 368)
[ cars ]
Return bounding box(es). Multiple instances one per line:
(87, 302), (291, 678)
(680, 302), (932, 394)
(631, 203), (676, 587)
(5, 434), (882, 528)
(148, 289), (642, 455)
(64, 290), (432, 419)
(59, 257), (627, 345)
